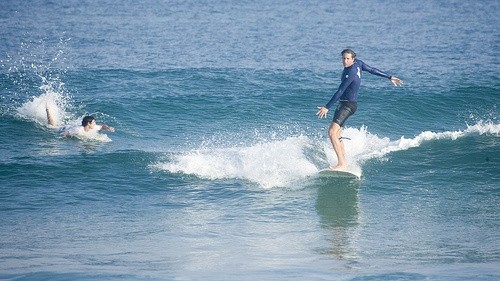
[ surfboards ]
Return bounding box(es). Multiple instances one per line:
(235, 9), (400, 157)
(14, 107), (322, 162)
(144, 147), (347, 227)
(318, 167), (361, 179)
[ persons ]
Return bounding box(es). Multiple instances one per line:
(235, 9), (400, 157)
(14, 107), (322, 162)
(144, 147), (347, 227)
(45, 98), (114, 139)
(315, 49), (403, 170)
